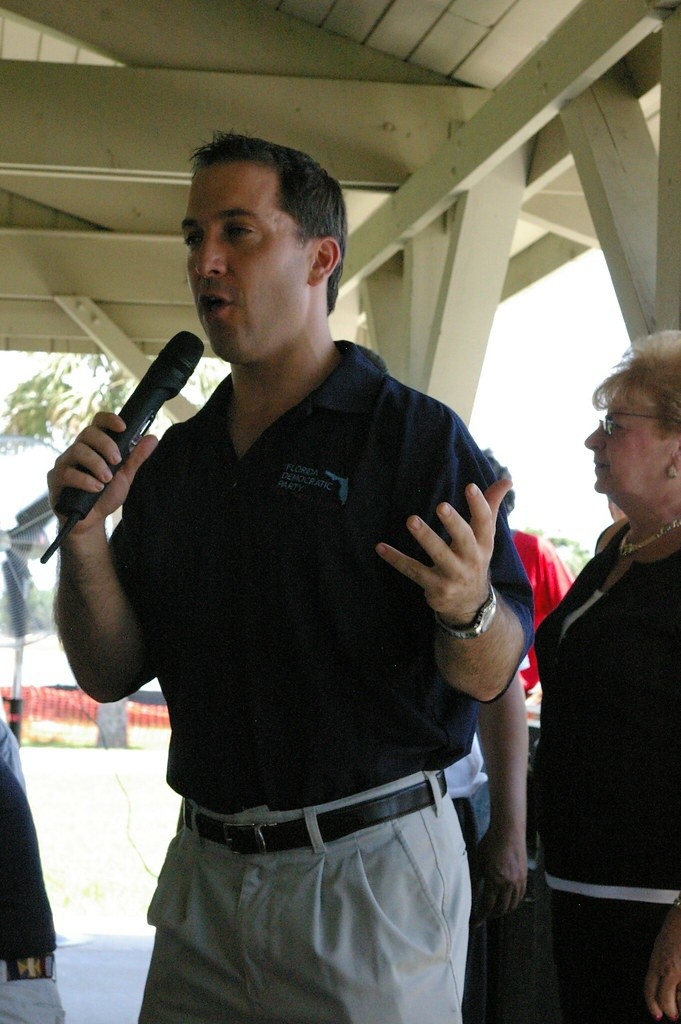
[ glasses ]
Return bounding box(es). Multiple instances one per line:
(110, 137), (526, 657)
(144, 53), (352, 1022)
(599, 412), (665, 435)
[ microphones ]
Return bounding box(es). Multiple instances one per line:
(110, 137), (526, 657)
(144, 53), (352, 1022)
(40, 331), (204, 563)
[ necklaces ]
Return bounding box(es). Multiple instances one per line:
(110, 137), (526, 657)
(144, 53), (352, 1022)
(620, 519), (681, 555)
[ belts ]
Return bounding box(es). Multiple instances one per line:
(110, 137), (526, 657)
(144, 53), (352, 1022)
(180, 770), (447, 853)
(7, 953), (54, 980)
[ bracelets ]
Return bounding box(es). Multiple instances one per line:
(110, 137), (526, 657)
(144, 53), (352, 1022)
(673, 898), (681, 909)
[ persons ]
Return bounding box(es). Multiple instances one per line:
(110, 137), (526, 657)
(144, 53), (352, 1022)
(47, 131), (530, 1024)
(445, 448), (628, 1024)
(532, 331), (681, 1024)
(0, 764), (64, 1024)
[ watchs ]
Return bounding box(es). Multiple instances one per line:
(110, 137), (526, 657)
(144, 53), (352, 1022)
(432, 584), (498, 640)
